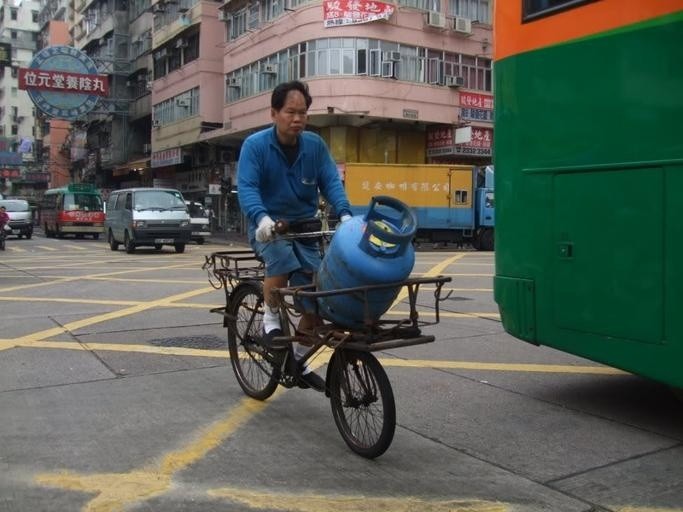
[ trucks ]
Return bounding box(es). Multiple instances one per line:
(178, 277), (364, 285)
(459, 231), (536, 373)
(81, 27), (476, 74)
(344, 162), (495, 251)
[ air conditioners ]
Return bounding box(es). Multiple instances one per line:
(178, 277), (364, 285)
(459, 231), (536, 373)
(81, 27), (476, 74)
(259, 63), (276, 75)
(383, 51), (401, 63)
(425, 11), (472, 35)
(445, 76), (463, 87)
(225, 77), (241, 88)
(175, 98), (188, 107)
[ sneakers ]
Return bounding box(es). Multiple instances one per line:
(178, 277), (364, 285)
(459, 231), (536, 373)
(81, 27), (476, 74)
(257, 322), (288, 354)
(297, 370), (325, 392)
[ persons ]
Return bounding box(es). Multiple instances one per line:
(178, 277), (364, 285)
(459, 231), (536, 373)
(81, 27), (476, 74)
(203, 204), (215, 232)
(237, 80), (355, 390)
(0, 206), (10, 235)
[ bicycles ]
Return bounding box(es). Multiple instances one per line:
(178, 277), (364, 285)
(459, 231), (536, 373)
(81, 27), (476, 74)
(202, 213), (452, 461)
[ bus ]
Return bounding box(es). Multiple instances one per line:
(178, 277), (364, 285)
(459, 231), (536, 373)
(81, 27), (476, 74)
(40, 183), (107, 240)
(491, 0), (683, 391)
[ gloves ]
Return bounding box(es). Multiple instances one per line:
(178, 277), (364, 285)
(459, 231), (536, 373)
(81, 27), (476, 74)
(339, 213), (353, 224)
(253, 215), (276, 243)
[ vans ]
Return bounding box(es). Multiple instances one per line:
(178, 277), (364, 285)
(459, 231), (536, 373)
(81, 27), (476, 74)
(104, 187), (192, 254)
(184, 199), (212, 245)
(0, 200), (33, 239)
